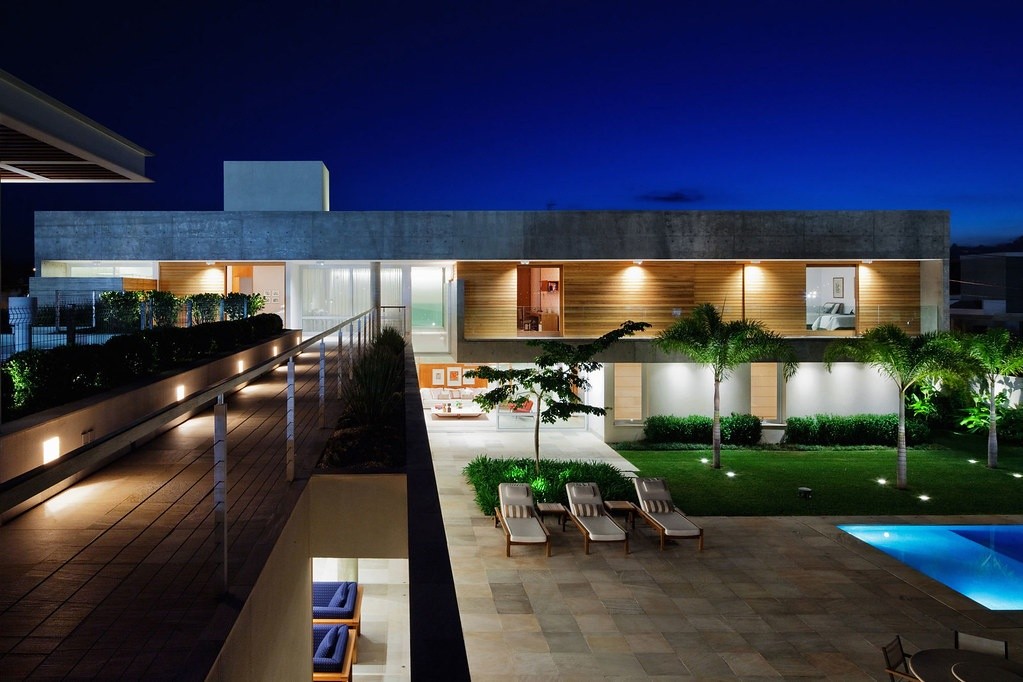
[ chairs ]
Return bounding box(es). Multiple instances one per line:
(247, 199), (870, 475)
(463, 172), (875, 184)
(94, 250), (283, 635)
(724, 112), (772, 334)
(881, 634), (921, 682)
(494, 482), (553, 558)
(953, 630), (1008, 659)
(562, 481), (630, 557)
(631, 476), (704, 554)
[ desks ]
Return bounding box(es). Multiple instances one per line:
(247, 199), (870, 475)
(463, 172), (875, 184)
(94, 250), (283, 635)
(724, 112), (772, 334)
(538, 311), (561, 333)
(909, 647), (1023, 682)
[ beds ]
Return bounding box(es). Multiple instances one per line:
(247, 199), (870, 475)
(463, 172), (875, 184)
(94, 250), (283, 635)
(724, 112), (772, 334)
(811, 313), (856, 332)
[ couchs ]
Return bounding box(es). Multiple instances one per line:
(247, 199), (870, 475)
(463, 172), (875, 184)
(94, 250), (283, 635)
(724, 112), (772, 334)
(509, 399), (534, 413)
(311, 581), (364, 682)
(806, 306), (843, 329)
(420, 387), (488, 409)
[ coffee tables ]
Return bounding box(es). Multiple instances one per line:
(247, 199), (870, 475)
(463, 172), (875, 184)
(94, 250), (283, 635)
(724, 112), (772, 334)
(537, 502), (566, 533)
(604, 500), (636, 532)
(431, 406), (487, 417)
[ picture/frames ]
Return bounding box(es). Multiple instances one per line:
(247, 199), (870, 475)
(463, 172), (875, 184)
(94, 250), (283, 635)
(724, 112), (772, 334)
(833, 277), (844, 298)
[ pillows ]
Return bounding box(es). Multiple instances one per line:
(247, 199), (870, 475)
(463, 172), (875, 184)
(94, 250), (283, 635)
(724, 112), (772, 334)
(422, 389), (472, 400)
(822, 302), (841, 314)
(328, 581), (348, 607)
(314, 625), (339, 659)
